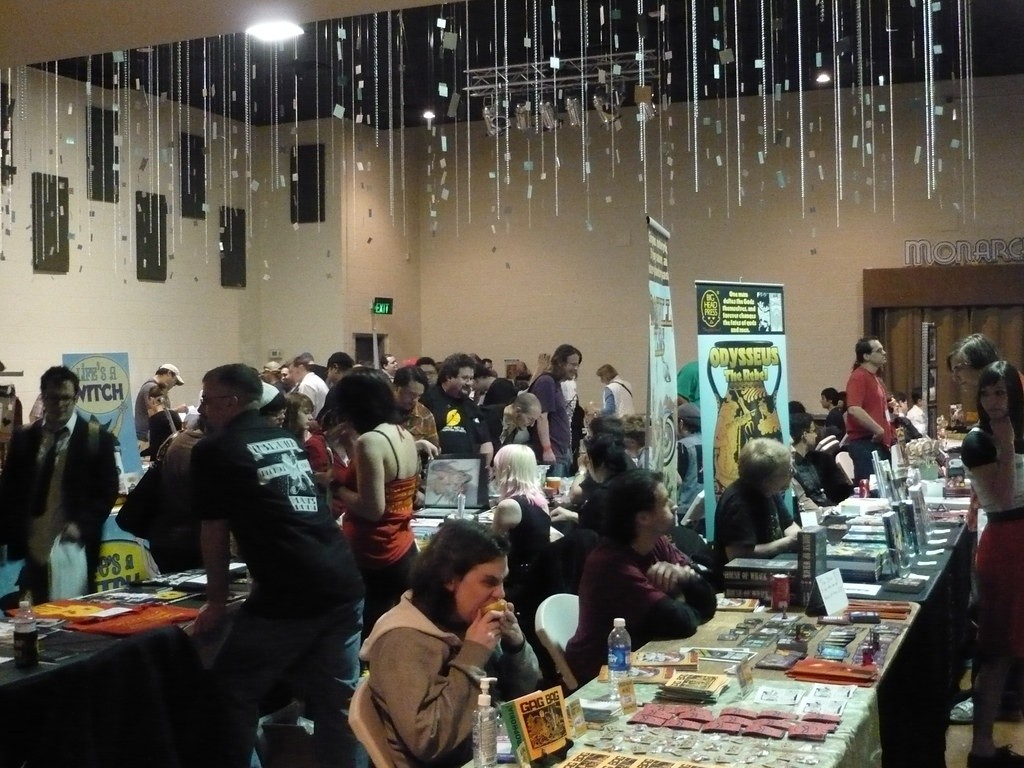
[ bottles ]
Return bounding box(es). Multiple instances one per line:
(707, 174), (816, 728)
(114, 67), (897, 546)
(13, 601), (39, 672)
(608, 618), (632, 698)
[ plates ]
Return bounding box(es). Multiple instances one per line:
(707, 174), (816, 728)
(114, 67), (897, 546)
(35, 616), (67, 629)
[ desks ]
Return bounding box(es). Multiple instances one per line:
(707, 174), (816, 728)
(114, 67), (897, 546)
(0, 421), (976, 768)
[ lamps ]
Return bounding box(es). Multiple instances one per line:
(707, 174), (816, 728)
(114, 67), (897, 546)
(476, 79), (661, 136)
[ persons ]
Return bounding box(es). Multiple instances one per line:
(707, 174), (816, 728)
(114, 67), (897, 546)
(115, 335), (922, 592)
(563, 467), (717, 691)
(0, 365), (118, 607)
(312, 366), (419, 646)
(944, 332), (1024, 722)
(960, 362), (1024, 768)
(194, 363), (371, 768)
(357, 520), (540, 768)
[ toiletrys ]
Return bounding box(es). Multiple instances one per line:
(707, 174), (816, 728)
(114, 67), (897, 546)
(471, 676), (498, 768)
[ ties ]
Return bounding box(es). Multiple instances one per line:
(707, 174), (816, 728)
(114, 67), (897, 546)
(35, 424), (67, 518)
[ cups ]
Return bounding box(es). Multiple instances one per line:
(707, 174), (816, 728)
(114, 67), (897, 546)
(489, 494), (500, 507)
(542, 477), (562, 506)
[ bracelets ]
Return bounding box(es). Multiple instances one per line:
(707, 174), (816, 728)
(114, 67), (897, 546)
(329, 480), (343, 496)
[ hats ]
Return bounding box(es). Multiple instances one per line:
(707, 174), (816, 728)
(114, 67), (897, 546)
(677, 402), (700, 419)
(161, 363), (186, 387)
(256, 382), (286, 413)
(264, 359), (281, 375)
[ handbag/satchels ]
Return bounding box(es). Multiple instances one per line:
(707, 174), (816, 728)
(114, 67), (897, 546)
(48, 531), (87, 600)
(523, 421), (541, 459)
(118, 430), (179, 539)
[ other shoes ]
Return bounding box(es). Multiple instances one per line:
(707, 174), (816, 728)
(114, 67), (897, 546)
(949, 698), (975, 724)
(966, 744), (1024, 768)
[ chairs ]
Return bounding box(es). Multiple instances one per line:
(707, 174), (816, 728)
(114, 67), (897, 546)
(346, 670), (391, 768)
(39, 631), (221, 768)
(820, 438), (840, 452)
(680, 489), (705, 527)
(816, 434), (837, 449)
(534, 592), (579, 691)
(672, 526), (715, 563)
(834, 451), (856, 486)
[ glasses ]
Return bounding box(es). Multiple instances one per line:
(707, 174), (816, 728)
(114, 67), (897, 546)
(45, 391), (78, 407)
(264, 409), (289, 421)
(200, 392), (238, 405)
